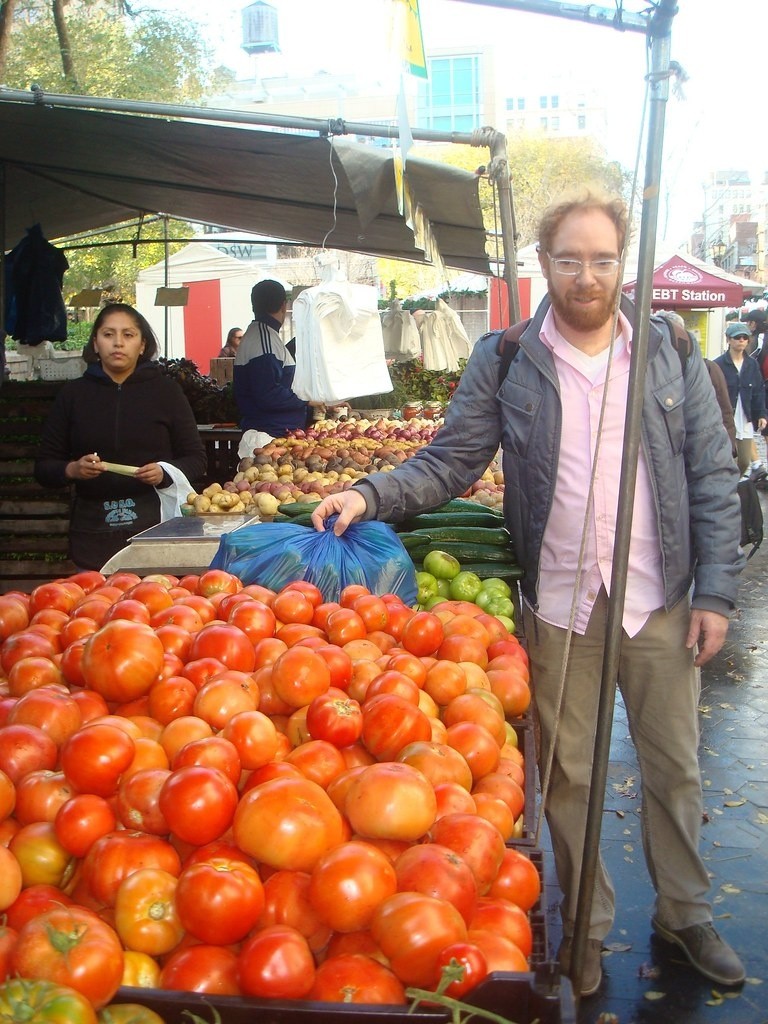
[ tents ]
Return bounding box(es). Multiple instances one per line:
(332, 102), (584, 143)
(514, 236), (761, 358)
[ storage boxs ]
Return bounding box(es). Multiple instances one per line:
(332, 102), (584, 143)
(114, 594), (576, 1024)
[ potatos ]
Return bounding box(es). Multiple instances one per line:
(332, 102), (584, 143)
(180, 437), (505, 511)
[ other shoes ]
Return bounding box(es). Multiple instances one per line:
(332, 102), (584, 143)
(752, 465), (768, 490)
(556, 935), (602, 996)
(651, 910), (747, 986)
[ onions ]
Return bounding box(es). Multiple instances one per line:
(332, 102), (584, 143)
(284, 416), (446, 442)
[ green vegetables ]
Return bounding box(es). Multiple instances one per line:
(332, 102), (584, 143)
(0, 374), (83, 564)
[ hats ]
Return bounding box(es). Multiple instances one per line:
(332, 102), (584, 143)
(726, 323), (752, 337)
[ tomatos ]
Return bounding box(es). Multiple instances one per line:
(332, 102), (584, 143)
(0, 551), (544, 1024)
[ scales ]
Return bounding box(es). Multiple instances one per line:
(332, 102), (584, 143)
(98, 515), (264, 575)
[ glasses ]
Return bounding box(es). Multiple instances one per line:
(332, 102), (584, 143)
(543, 249), (625, 277)
(733, 335), (750, 340)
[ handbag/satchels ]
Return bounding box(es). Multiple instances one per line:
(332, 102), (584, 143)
(151, 464), (196, 521)
(737, 480), (764, 561)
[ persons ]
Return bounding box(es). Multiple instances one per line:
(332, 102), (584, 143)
(310, 186), (747, 996)
(233, 279), (352, 455)
(219, 328), (242, 357)
(713, 310), (768, 492)
(36, 304), (208, 573)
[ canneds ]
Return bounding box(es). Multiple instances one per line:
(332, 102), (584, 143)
(402, 400), (453, 421)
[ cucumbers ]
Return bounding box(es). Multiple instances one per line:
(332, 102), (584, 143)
(274, 496), (524, 582)
